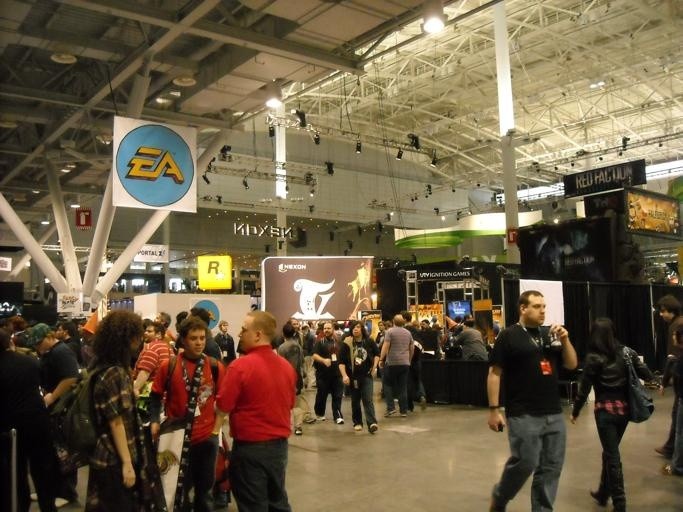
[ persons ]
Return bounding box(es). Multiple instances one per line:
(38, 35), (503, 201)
(487, 289), (579, 512)
(569, 316), (664, 512)
(651, 294), (683, 477)
(0, 303), (297, 512)
(272, 310), (486, 433)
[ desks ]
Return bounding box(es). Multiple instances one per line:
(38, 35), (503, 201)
(419, 359), (504, 409)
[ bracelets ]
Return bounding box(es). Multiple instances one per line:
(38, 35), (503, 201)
(488, 404), (501, 410)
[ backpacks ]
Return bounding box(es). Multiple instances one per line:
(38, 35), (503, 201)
(45, 351), (122, 471)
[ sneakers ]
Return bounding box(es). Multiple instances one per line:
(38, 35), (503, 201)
(354, 425), (363, 431)
(590, 492), (608, 507)
(653, 445), (672, 458)
(314, 416), (325, 421)
(662, 463), (674, 477)
(335, 418), (345, 424)
(294, 426), (302, 434)
(490, 487), (507, 511)
(369, 423), (378, 433)
(303, 417), (315, 423)
(385, 398), (429, 417)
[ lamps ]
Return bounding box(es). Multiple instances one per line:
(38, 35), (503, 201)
(69, 195), (81, 208)
(40, 213), (50, 224)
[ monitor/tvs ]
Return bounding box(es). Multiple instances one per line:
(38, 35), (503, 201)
(447, 300), (473, 321)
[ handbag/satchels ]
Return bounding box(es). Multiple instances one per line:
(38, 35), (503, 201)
(619, 348), (655, 424)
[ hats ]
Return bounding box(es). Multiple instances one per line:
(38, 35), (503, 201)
(24, 323), (52, 348)
